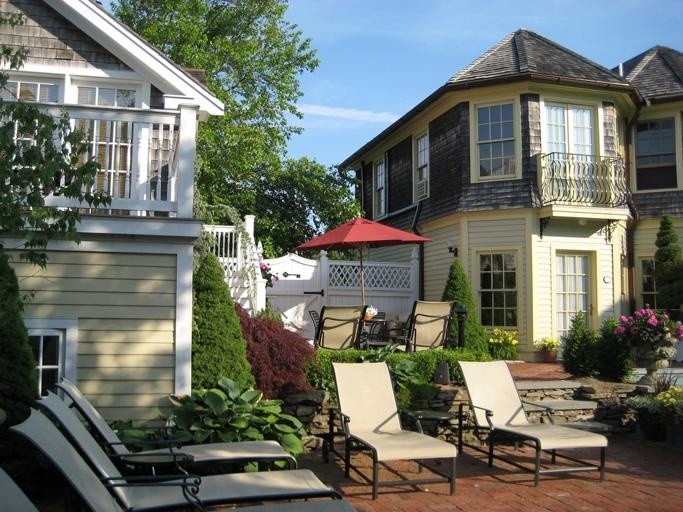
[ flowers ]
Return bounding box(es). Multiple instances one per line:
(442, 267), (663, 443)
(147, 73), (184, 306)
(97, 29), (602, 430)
(532, 337), (561, 352)
(652, 384), (683, 415)
(613, 308), (683, 345)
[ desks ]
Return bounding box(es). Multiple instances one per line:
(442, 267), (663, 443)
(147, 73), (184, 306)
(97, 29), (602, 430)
(398, 409), (456, 472)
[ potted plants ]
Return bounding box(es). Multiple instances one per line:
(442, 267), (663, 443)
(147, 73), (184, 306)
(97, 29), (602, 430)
(625, 391), (669, 442)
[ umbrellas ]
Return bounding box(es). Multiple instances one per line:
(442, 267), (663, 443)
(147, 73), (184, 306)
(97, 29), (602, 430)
(291, 213), (433, 307)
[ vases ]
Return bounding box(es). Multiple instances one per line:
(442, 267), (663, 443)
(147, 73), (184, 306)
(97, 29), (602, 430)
(666, 415), (682, 425)
(630, 345), (677, 393)
(544, 349), (557, 363)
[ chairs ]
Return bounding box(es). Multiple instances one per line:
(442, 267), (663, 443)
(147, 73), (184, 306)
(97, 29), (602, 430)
(35, 390), (334, 511)
(309, 300), (459, 352)
(322, 361), (458, 500)
(460, 361), (609, 485)
(56, 380), (298, 478)
(1, 468), (360, 512)
